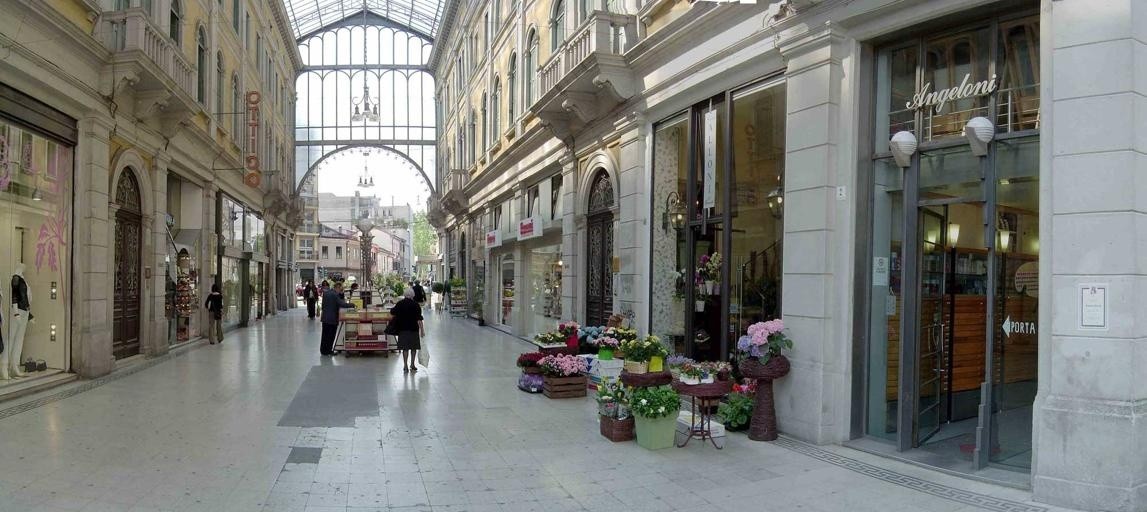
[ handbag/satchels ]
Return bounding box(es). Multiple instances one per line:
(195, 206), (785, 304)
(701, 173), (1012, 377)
(384, 316), (398, 336)
(418, 342), (429, 368)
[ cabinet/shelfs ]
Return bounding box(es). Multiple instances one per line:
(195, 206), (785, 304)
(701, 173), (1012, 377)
(175, 266), (201, 342)
(450, 284), (467, 318)
(331, 286), (402, 356)
(889, 240), (996, 298)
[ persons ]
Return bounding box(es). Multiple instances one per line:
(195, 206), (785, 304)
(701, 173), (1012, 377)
(411, 279), (427, 318)
(0, 262), (32, 382)
(302, 276), (359, 321)
(224, 280), (257, 323)
(389, 287), (425, 372)
(319, 281), (358, 356)
(409, 281), (413, 289)
(204, 283), (224, 344)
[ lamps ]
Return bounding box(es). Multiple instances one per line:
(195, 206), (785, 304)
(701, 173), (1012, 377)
(31, 167), (53, 203)
(886, 127), (918, 169)
(346, 1), (386, 126)
(766, 167), (784, 221)
(663, 191), (687, 237)
(963, 113), (997, 160)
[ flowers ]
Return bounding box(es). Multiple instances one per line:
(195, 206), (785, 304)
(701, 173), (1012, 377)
(513, 241), (794, 451)
